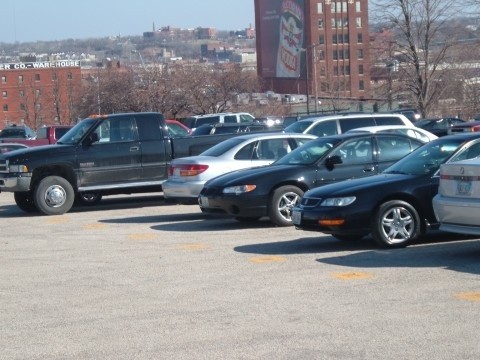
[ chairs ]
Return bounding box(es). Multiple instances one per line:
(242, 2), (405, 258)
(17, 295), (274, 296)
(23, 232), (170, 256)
(262, 151), (275, 159)
(277, 148), (287, 157)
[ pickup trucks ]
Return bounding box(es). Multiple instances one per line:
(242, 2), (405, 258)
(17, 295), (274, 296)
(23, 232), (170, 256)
(0, 124), (73, 154)
(0, 111), (254, 215)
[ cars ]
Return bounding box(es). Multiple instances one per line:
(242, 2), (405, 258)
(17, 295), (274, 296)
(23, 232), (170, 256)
(198, 132), (454, 227)
(432, 158), (480, 236)
(163, 109), (480, 157)
(289, 131), (480, 250)
(0, 124), (35, 138)
(162, 131), (348, 199)
